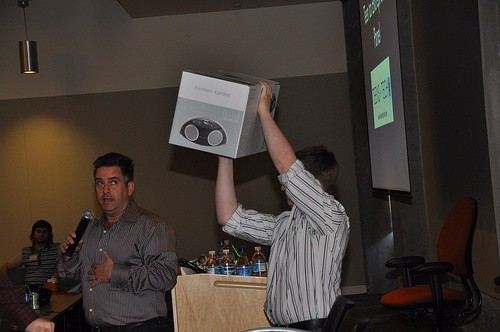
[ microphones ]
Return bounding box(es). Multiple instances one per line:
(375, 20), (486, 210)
(64, 209), (95, 257)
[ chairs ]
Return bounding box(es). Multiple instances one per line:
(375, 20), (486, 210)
(172, 267), (312, 332)
(380, 197), (483, 332)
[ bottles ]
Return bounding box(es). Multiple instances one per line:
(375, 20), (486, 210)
(221, 250), (235, 275)
(206, 250), (221, 274)
(220, 240), (234, 261)
(252, 246), (267, 277)
(235, 252), (252, 276)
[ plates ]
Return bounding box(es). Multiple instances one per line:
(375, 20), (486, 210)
(189, 258), (207, 270)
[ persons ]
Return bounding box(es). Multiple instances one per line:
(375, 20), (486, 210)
(61, 150), (180, 332)
(215, 81), (350, 331)
(20, 220), (59, 288)
(0, 270), (55, 332)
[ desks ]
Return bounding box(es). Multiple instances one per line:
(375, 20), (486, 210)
(9, 292), (83, 332)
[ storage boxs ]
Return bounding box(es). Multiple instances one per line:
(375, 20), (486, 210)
(169, 70), (281, 160)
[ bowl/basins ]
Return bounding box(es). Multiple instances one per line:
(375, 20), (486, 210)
(23, 275), (69, 306)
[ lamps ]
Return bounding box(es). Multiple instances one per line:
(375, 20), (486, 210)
(18, 0), (39, 75)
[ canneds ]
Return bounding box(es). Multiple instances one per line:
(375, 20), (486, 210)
(29, 291), (39, 309)
